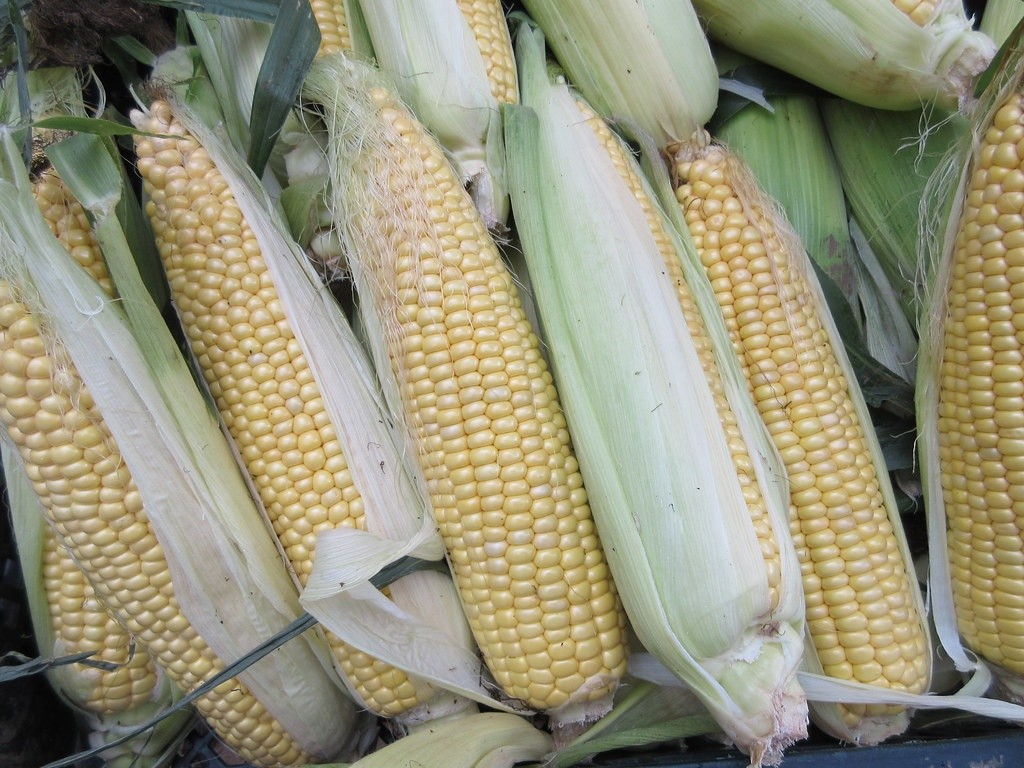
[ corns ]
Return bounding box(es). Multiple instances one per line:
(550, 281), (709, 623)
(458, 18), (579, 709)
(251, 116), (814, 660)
(0, 0), (1024, 768)
